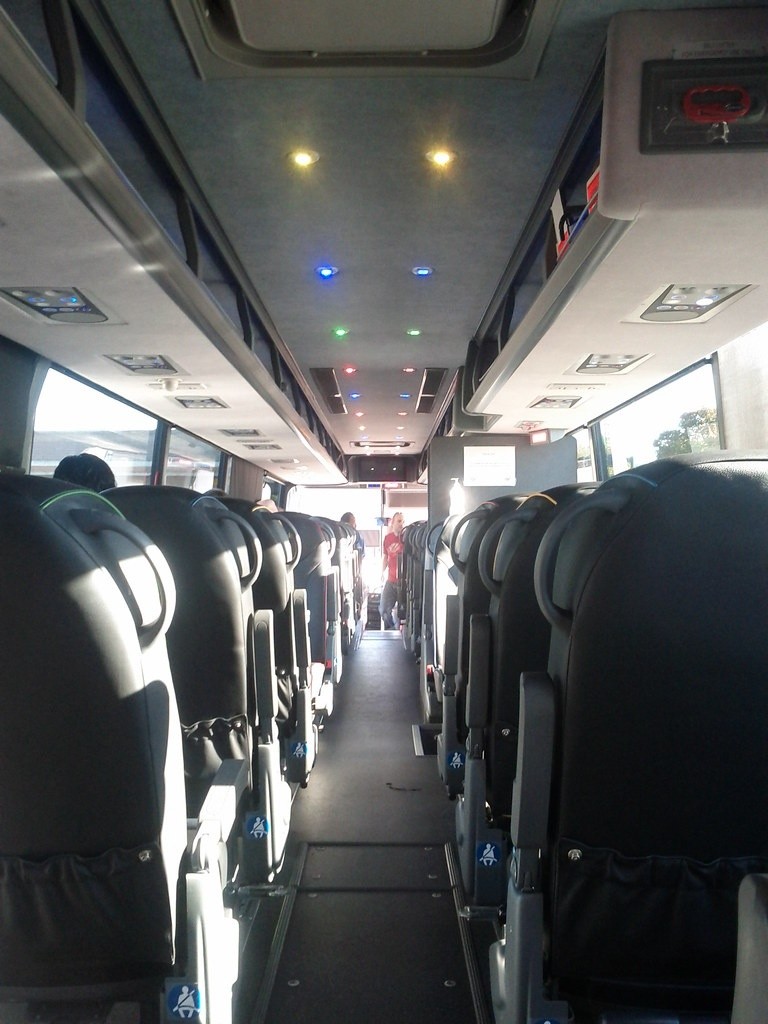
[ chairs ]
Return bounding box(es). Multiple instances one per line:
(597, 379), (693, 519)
(0, 472), (368, 1024)
(395, 448), (768, 1024)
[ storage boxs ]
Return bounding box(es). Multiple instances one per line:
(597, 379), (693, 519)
(587, 164), (604, 214)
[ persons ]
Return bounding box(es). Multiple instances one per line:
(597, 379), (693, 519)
(54, 452), (113, 493)
(377, 512), (408, 630)
(341, 511), (364, 567)
